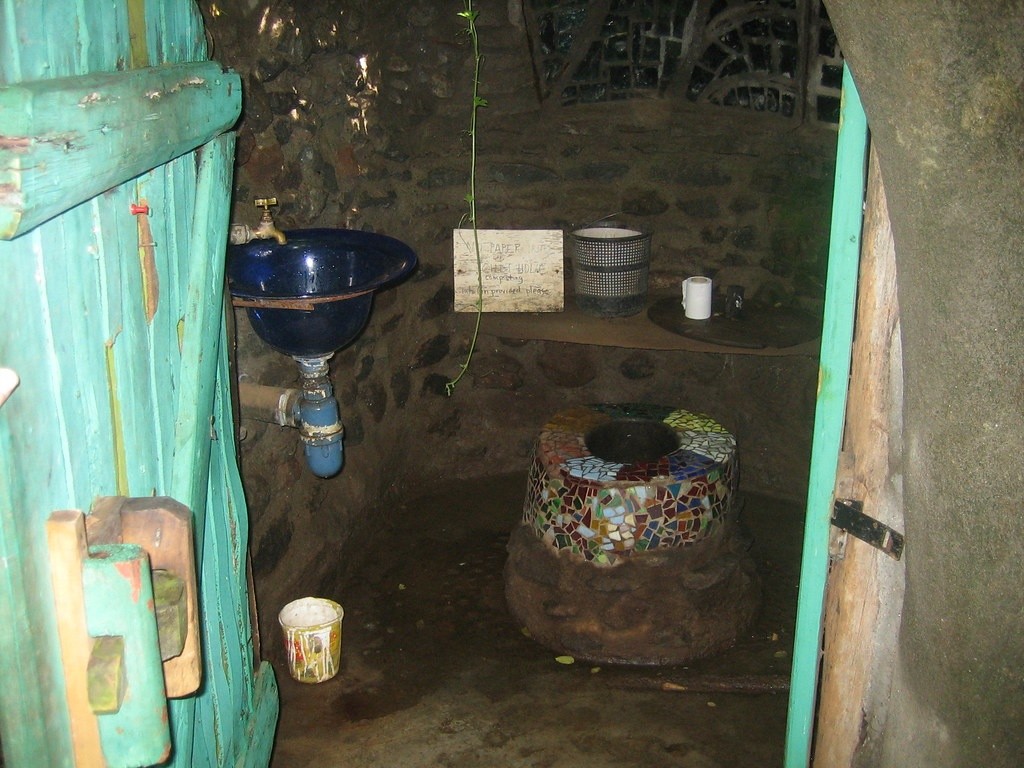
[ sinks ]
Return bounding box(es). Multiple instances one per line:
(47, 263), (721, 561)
(225, 228), (417, 357)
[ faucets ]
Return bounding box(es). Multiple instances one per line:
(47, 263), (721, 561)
(246, 195), (288, 246)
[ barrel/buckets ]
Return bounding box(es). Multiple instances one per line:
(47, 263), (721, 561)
(567, 213), (653, 313)
(277, 597), (343, 683)
